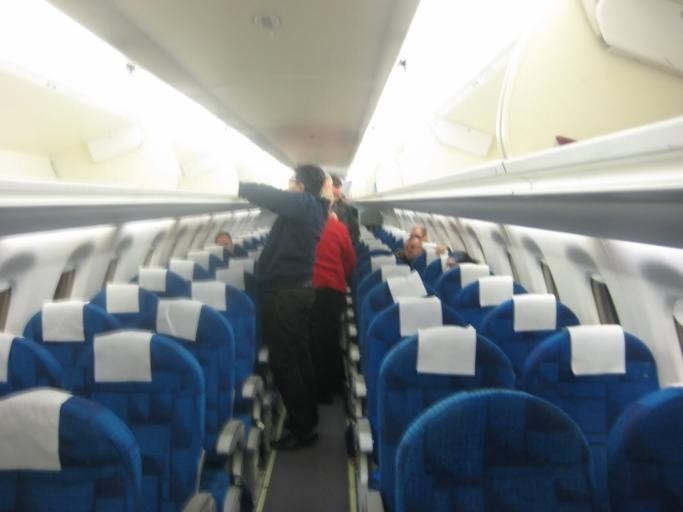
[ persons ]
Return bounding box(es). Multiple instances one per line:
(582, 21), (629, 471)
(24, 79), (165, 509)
(394, 226), (474, 276)
(214, 231), (236, 258)
(311, 210), (358, 402)
(238, 164), (329, 450)
(327, 174), (360, 247)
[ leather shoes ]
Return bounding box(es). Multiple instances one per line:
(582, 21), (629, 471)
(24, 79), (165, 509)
(269, 430), (321, 451)
